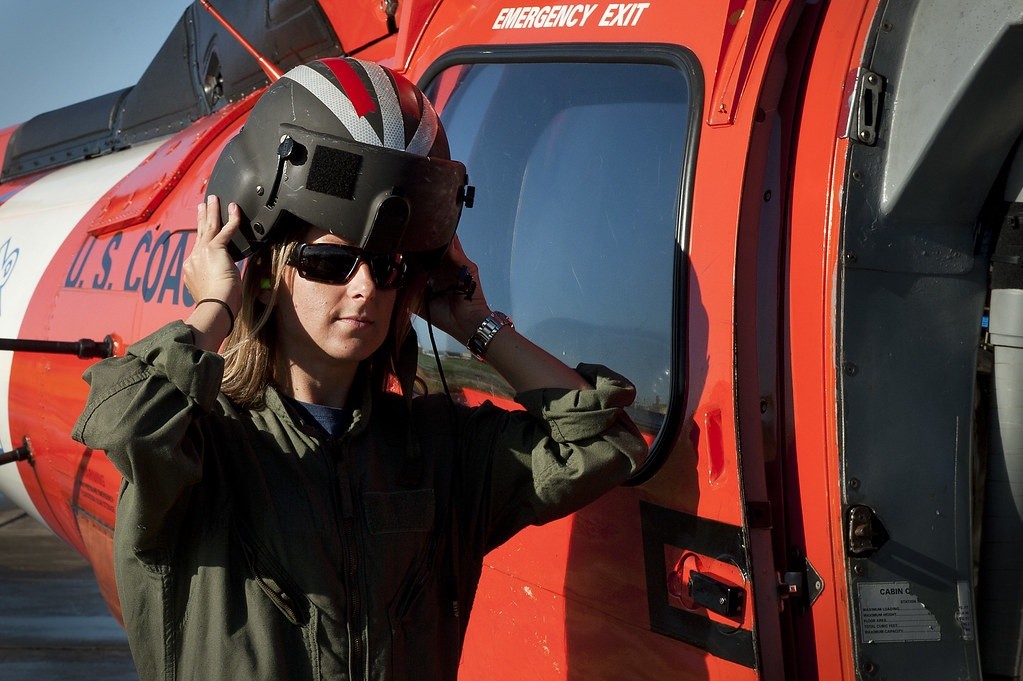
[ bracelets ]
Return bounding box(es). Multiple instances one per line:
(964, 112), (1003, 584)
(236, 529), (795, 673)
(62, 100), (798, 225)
(195, 298), (235, 338)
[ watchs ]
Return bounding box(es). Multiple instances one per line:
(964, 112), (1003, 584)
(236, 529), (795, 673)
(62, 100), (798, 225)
(465, 310), (515, 361)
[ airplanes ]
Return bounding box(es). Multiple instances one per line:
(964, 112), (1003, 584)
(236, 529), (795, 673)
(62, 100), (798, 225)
(0, 1), (1020, 681)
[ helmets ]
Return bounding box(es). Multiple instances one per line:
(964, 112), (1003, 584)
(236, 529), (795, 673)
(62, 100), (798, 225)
(199, 56), (476, 278)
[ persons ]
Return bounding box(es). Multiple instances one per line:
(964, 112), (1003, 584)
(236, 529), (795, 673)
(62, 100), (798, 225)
(71, 57), (650, 681)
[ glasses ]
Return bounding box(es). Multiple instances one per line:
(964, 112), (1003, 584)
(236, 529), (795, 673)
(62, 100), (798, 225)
(284, 241), (409, 290)
